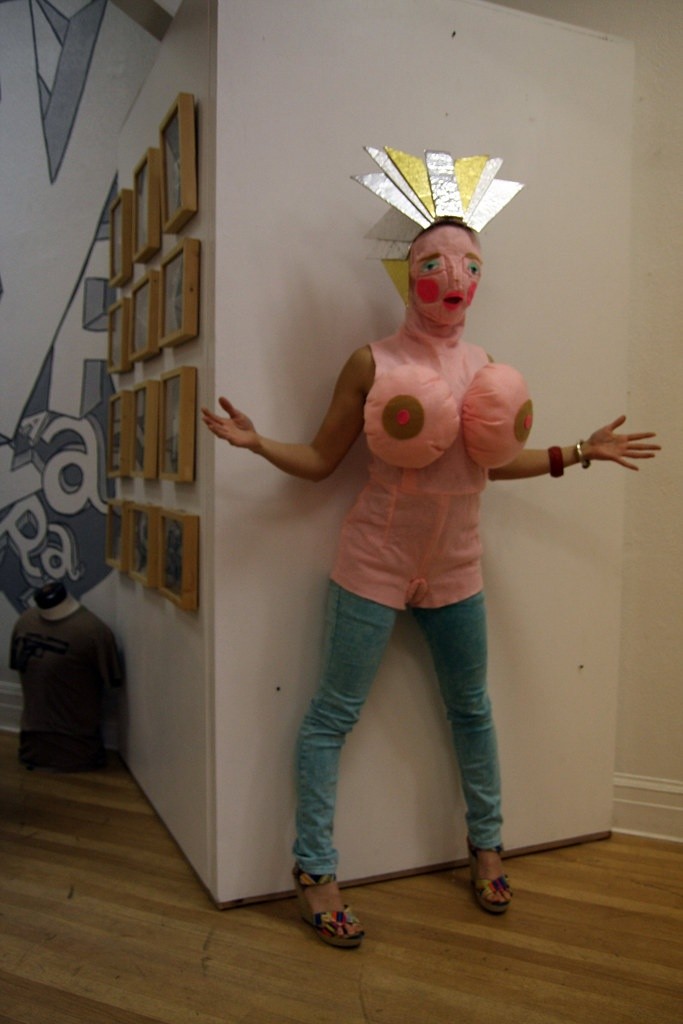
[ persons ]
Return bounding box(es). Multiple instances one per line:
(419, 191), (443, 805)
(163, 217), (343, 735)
(9, 581), (126, 774)
(202, 219), (663, 950)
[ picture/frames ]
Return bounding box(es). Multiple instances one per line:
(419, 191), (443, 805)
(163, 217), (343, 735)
(155, 508), (198, 613)
(158, 366), (198, 483)
(107, 188), (131, 291)
(105, 296), (132, 374)
(106, 389), (131, 479)
(125, 501), (163, 590)
(128, 378), (158, 484)
(128, 268), (159, 363)
(131, 146), (161, 264)
(158, 91), (197, 234)
(105, 498), (135, 575)
(156, 235), (200, 352)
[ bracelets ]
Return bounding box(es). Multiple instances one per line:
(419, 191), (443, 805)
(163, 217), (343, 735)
(547, 447), (564, 477)
(575, 440), (590, 469)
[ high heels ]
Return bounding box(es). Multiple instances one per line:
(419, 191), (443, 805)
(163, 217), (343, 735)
(291, 861), (366, 947)
(466, 836), (514, 912)
